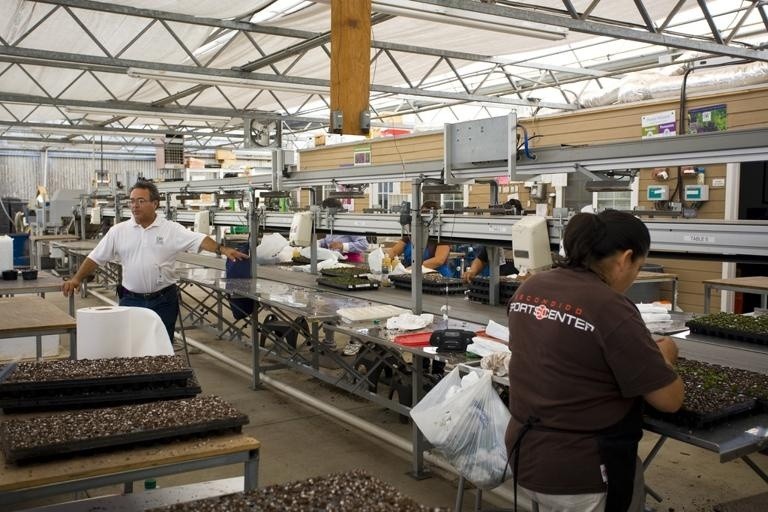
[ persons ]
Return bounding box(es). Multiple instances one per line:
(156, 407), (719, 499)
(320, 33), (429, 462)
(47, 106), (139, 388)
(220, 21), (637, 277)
(504, 207), (685, 512)
(59, 180), (250, 349)
(460, 198), (528, 285)
(382, 199), (452, 391)
(316, 197), (370, 356)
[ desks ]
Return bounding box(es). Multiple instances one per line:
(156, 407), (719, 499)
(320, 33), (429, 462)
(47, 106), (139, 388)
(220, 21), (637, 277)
(0, 266), (766, 511)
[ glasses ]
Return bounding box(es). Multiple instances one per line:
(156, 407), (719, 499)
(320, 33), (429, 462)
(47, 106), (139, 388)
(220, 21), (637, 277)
(130, 199), (153, 204)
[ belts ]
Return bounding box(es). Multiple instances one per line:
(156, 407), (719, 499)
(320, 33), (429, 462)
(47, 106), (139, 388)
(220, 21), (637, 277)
(124, 282), (176, 300)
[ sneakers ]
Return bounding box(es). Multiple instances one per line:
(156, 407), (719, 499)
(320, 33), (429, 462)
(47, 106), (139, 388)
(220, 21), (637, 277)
(312, 340), (362, 356)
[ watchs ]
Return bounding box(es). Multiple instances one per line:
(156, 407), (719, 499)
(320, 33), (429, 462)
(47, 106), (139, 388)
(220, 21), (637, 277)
(215, 243), (223, 256)
